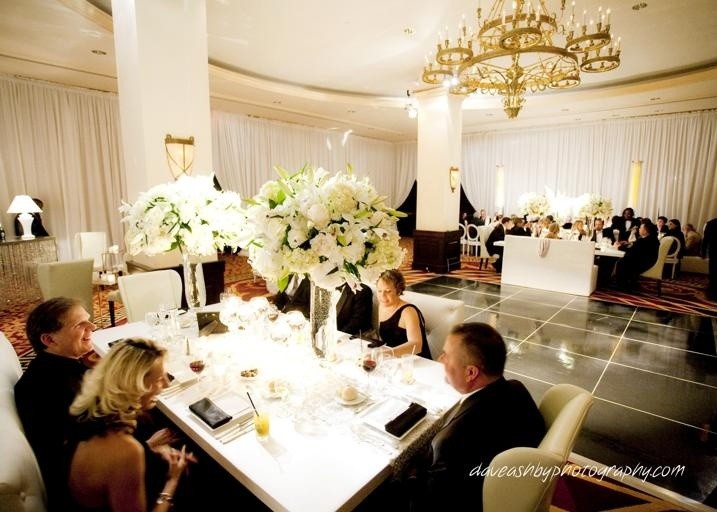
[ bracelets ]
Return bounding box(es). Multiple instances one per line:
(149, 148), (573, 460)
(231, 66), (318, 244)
(154, 492), (176, 507)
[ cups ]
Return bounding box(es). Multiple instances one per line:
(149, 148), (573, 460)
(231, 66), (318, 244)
(343, 378), (356, 400)
(403, 354), (413, 381)
(254, 412), (271, 435)
(145, 301), (181, 341)
(371, 350), (383, 370)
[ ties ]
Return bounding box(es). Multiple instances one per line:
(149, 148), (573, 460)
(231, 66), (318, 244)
(290, 274), (299, 298)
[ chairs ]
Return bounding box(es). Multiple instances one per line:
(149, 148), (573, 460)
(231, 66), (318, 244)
(456, 216), (682, 297)
(80, 231), (107, 270)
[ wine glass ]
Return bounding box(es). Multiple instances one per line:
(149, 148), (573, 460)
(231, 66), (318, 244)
(189, 355), (207, 393)
(364, 356), (376, 382)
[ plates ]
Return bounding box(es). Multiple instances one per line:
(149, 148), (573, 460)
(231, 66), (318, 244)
(360, 398), (428, 442)
(336, 392), (367, 406)
(189, 393), (255, 431)
(240, 368), (262, 381)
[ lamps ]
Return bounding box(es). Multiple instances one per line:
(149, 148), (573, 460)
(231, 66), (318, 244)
(5, 194), (43, 240)
(413, 0), (620, 121)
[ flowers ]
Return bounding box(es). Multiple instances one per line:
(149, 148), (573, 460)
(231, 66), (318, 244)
(114, 176), (261, 305)
(249, 169), (409, 305)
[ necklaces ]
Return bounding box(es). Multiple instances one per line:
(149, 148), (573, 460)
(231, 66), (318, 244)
(383, 299), (400, 316)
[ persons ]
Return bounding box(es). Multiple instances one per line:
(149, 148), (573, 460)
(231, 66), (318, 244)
(406, 321), (546, 512)
(46, 334), (198, 512)
(272, 262), (309, 316)
(465, 207), (717, 283)
(332, 280), (371, 333)
(374, 267), (430, 363)
(15, 197), (50, 237)
(12, 296), (185, 481)
